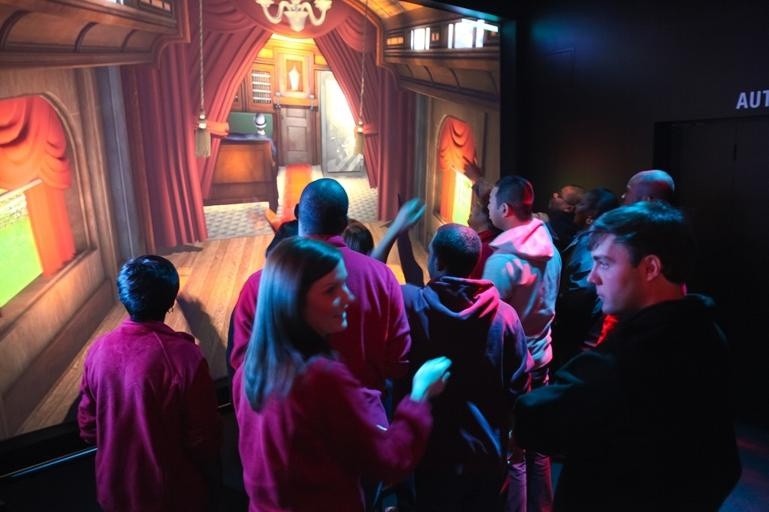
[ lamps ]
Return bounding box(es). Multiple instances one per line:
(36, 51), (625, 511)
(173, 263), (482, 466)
(256, 0), (332, 32)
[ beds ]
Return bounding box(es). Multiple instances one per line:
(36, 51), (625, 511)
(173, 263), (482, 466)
(204, 111), (278, 215)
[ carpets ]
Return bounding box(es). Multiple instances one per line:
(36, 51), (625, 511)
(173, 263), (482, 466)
(277, 163), (324, 231)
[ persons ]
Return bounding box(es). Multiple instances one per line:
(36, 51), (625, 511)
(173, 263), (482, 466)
(224, 165), (746, 511)
(75, 254), (223, 511)
